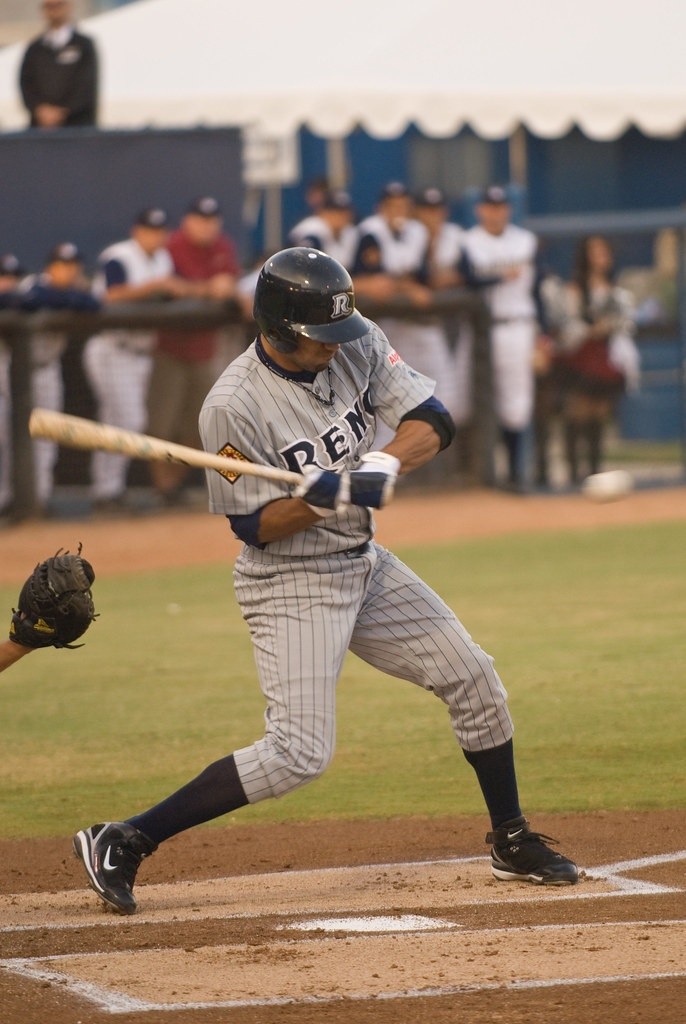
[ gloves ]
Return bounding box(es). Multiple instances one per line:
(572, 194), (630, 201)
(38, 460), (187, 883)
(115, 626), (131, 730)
(356, 451), (401, 513)
(302, 473), (351, 519)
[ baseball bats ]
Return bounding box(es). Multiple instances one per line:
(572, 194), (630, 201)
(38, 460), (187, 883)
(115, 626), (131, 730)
(29, 403), (303, 484)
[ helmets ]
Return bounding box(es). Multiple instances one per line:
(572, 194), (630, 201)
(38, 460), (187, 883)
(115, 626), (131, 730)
(250, 245), (370, 353)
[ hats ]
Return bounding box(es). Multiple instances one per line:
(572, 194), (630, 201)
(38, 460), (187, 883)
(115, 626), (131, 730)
(1, 255), (25, 275)
(382, 184), (406, 199)
(49, 242), (81, 261)
(326, 186), (350, 209)
(415, 189), (446, 207)
(478, 186), (507, 205)
(192, 197), (222, 215)
(136, 206), (167, 227)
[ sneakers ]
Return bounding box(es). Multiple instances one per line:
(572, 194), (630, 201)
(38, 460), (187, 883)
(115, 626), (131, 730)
(485, 821), (579, 885)
(71, 821), (158, 915)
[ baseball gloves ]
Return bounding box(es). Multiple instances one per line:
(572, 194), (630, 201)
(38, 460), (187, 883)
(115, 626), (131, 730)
(8, 538), (102, 651)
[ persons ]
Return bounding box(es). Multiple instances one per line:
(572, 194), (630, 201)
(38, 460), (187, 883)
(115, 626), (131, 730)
(19, 0), (98, 131)
(0, 554), (95, 680)
(72, 247), (578, 915)
(0, 177), (683, 516)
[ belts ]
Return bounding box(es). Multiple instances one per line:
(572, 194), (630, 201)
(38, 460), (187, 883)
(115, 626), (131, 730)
(343, 541), (369, 558)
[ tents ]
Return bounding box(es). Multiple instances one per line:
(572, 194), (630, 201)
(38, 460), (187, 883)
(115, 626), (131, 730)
(0, 0), (684, 261)
